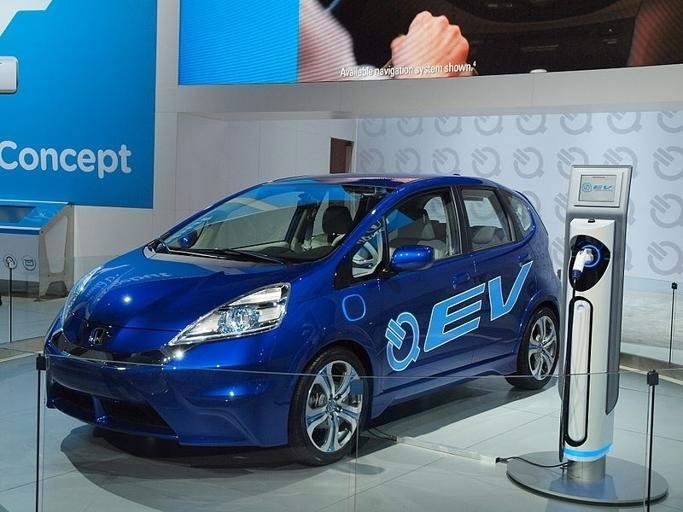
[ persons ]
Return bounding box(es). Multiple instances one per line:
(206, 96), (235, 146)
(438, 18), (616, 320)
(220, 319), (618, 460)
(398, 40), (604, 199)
(299, 0), (473, 83)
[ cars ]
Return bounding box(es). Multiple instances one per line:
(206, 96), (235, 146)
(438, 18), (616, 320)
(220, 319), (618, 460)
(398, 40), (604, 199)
(42, 173), (562, 468)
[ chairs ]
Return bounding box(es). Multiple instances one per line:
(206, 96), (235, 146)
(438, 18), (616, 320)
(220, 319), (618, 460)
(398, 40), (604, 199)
(389, 210), (446, 260)
(470, 226), (506, 252)
(310, 205), (354, 248)
